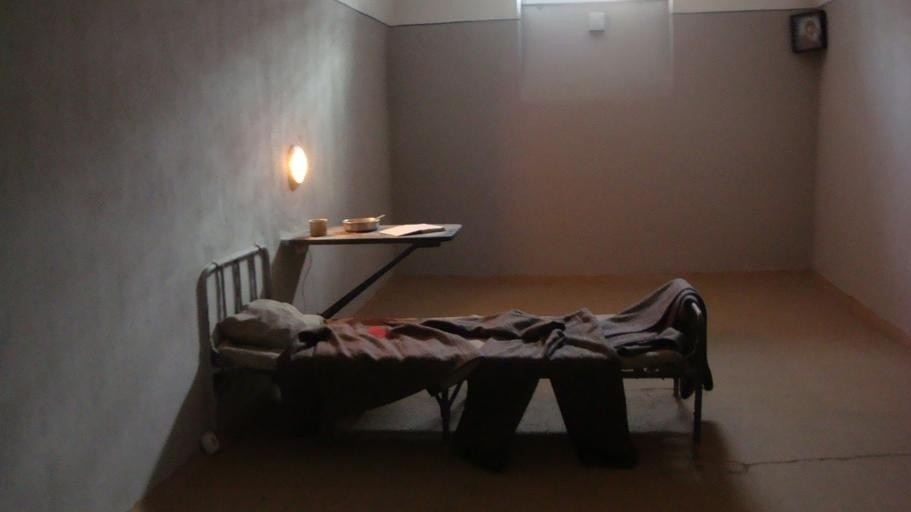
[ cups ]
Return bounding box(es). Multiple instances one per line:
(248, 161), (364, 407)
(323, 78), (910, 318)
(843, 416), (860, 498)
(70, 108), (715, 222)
(309, 218), (328, 237)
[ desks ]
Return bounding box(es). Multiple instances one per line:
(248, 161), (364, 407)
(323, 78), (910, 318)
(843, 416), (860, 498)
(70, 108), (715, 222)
(285, 222), (465, 321)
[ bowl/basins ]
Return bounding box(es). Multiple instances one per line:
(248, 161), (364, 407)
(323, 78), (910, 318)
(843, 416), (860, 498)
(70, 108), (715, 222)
(343, 217), (380, 232)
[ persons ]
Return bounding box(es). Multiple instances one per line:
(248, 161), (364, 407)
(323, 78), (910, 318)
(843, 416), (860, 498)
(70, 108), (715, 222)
(799, 17), (822, 47)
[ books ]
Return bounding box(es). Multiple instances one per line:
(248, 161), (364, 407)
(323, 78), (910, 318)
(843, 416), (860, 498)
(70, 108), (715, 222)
(381, 221), (447, 236)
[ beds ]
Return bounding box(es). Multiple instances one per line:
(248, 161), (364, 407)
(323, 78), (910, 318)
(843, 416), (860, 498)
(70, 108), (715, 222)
(197, 242), (712, 475)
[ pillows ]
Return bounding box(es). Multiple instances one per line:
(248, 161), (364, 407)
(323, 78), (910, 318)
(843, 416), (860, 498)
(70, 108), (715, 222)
(217, 296), (329, 353)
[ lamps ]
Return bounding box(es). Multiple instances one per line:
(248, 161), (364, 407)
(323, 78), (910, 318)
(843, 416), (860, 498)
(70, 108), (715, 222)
(283, 142), (310, 186)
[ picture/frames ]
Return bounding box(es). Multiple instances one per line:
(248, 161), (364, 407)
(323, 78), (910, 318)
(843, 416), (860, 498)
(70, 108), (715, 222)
(787, 7), (829, 55)
